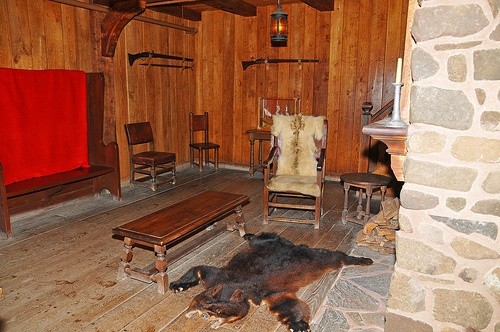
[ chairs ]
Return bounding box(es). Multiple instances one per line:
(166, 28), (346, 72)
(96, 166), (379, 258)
(189, 112), (219, 171)
(262, 114), (327, 230)
(246, 98), (301, 177)
(125, 122), (176, 190)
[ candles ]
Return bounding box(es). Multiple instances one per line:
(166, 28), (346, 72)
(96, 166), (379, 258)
(395, 57), (403, 83)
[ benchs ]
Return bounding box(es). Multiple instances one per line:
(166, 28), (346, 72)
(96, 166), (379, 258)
(0, 67), (121, 238)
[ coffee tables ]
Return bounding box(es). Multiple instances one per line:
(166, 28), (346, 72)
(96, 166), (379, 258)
(341, 172), (391, 226)
(113, 190), (250, 295)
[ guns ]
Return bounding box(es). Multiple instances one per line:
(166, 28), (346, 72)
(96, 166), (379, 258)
(128, 51), (194, 67)
(242, 58), (320, 70)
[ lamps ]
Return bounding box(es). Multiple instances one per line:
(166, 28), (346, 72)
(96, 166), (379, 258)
(271, 0), (289, 42)
